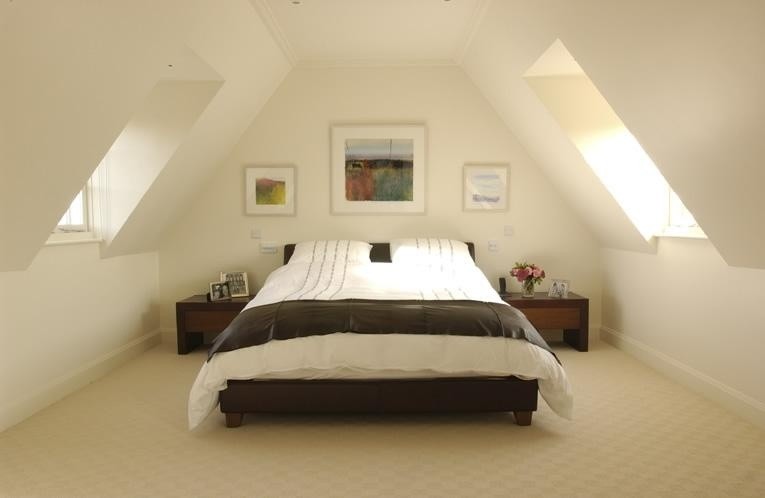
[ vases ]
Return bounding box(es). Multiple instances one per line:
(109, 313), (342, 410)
(522, 279), (535, 298)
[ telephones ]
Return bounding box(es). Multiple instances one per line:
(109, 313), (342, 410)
(499, 277), (512, 297)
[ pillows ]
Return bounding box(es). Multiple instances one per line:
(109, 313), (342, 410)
(287, 238), (374, 266)
(389, 238), (475, 267)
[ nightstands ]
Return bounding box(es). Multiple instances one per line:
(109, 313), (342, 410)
(175, 293), (253, 355)
(501, 287), (591, 354)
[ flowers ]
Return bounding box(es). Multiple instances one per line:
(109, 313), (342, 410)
(510, 259), (548, 294)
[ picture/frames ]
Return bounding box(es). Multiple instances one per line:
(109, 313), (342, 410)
(461, 161), (510, 213)
(329, 122), (428, 215)
(208, 270), (252, 302)
(244, 165), (297, 217)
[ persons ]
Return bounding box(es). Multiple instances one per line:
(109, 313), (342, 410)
(557, 282), (566, 298)
(213, 288), (223, 299)
(219, 283), (231, 297)
(549, 282), (562, 297)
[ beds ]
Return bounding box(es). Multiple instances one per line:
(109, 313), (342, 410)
(219, 239), (539, 428)
